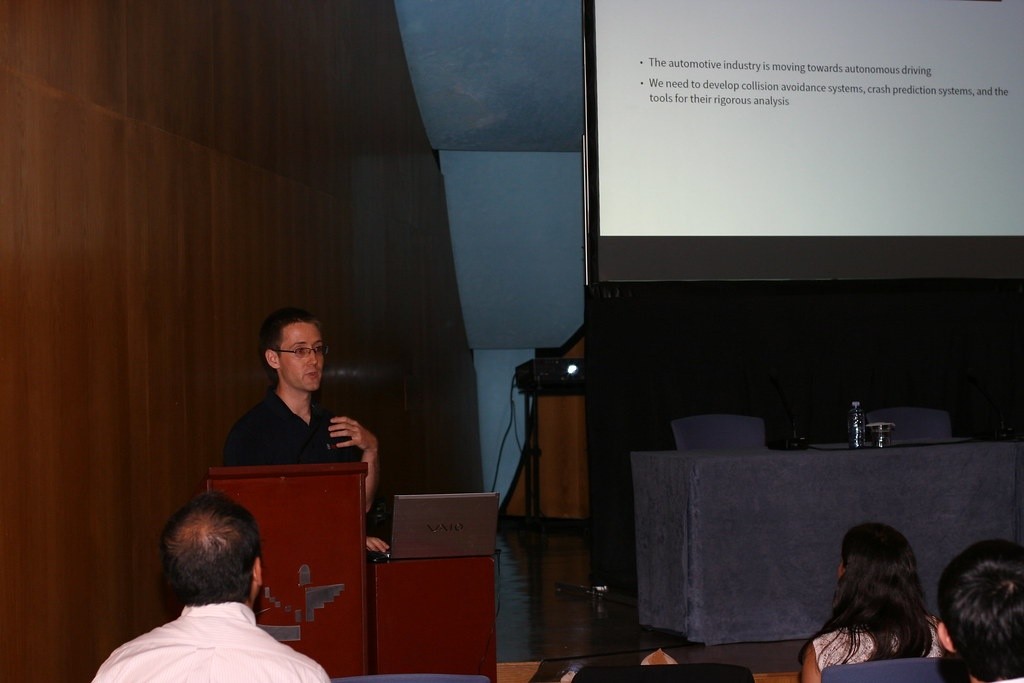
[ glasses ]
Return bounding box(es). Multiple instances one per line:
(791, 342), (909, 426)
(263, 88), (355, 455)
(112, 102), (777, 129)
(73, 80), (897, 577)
(273, 346), (328, 357)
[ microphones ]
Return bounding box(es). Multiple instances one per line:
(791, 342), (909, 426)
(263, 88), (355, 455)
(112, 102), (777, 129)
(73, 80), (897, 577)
(766, 365), (808, 450)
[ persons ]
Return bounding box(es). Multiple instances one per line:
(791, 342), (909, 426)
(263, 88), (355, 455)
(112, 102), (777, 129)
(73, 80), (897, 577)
(798, 523), (958, 683)
(91, 489), (332, 683)
(224, 306), (390, 554)
(937, 539), (1024, 683)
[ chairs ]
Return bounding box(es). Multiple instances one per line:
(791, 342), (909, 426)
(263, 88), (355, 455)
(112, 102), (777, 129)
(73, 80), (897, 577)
(318, 638), (964, 683)
(671, 415), (766, 450)
(866, 404), (954, 451)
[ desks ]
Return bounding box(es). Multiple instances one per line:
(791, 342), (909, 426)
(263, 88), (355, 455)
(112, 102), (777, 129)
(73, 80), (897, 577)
(630, 441), (1023, 646)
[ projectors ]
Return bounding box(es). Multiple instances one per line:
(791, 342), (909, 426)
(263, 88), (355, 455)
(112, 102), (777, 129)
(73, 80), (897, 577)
(515, 357), (585, 388)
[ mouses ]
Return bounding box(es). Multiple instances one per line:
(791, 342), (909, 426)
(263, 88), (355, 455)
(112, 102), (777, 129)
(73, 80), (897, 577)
(366, 551), (386, 563)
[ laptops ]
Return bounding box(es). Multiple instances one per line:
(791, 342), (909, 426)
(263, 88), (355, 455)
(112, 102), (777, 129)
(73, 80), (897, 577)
(381, 492), (500, 558)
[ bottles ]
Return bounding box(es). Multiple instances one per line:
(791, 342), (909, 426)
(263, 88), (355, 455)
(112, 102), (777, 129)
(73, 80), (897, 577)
(847, 401), (865, 449)
